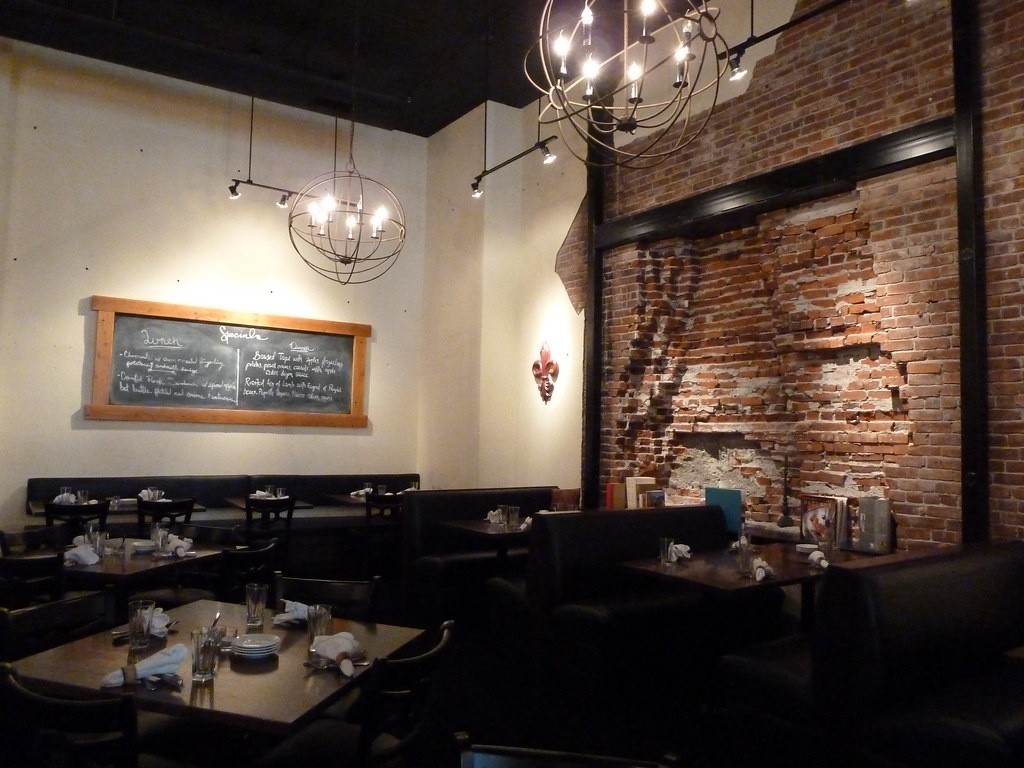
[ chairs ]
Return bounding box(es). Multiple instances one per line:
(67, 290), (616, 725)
(0, 521), (680, 768)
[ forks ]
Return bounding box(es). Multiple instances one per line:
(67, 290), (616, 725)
(306, 661), (369, 670)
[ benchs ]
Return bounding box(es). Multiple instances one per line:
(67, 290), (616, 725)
(392, 492), (1024, 766)
(27, 473), (422, 538)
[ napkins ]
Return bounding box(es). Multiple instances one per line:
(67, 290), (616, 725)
(519, 516), (532, 530)
(731, 540), (755, 551)
(273, 599), (331, 624)
(101, 643), (187, 686)
(309, 631), (366, 678)
(64, 545), (101, 566)
(483, 508), (504, 522)
(807, 550), (830, 568)
(666, 542), (690, 561)
(750, 557), (776, 581)
(54, 486), (374, 504)
(136, 605), (170, 638)
(166, 534), (193, 557)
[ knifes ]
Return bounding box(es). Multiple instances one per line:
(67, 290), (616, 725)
(112, 619), (180, 641)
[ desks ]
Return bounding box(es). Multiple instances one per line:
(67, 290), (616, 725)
(625, 543), (825, 658)
(364, 493), (401, 518)
(62, 535), (221, 624)
(244, 494), (297, 522)
(136, 496), (193, 534)
(435, 517), (531, 579)
(13, 598), (427, 768)
(42, 497), (110, 534)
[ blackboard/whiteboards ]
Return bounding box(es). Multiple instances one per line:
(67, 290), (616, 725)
(83, 295), (372, 428)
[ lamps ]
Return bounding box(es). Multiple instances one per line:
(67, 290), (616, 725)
(227, 85), (367, 227)
(717, 1), (847, 81)
(469, 28), (557, 199)
(284, 87), (405, 285)
(524, 0), (729, 170)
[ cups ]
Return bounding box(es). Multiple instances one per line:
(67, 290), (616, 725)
(191, 626), (221, 681)
(497, 505), (520, 529)
(244, 583), (267, 626)
(813, 533), (835, 558)
(84, 523), (107, 560)
(61, 486), (89, 504)
(410, 481), (419, 490)
(218, 626), (238, 651)
(659, 535), (683, 568)
(148, 487), (163, 502)
(149, 521), (170, 555)
(730, 537), (762, 575)
(265, 485), (287, 497)
(128, 601), (155, 650)
(306, 603), (333, 646)
(364, 483), (387, 496)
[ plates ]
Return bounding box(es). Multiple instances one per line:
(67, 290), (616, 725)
(232, 634), (280, 658)
(795, 543), (819, 554)
(132, 541), (157, 551)
